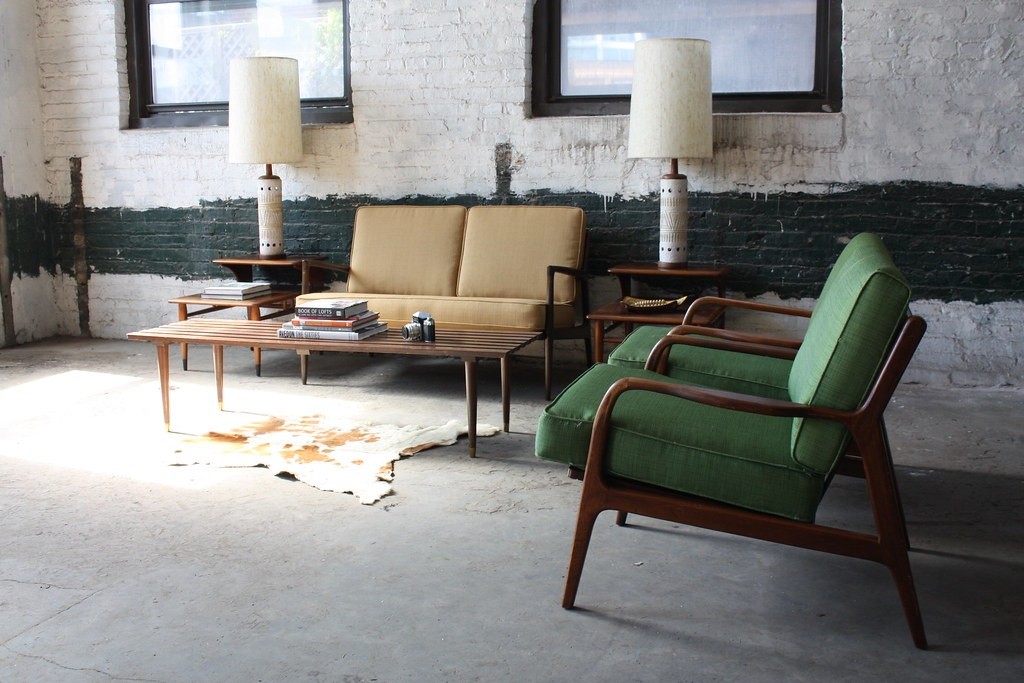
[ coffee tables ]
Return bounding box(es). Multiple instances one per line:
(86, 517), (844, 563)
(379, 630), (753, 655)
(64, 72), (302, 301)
(124, 316), (543, 461)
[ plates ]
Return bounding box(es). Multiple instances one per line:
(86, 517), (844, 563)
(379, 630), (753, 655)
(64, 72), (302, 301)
(620, 294), (689, 310)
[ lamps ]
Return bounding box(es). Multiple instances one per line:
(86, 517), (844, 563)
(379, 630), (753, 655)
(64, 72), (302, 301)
(622, 35), (719, 266)
(225, 51), (308, 258)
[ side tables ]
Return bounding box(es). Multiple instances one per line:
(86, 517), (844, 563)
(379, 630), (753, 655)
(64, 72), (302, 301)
(586, 262), (733, 367)
(168, 250), (351, 378)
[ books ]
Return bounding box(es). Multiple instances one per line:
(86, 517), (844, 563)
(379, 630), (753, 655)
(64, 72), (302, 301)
(201, 282), (272, 300)
(278, 300), (388, 342)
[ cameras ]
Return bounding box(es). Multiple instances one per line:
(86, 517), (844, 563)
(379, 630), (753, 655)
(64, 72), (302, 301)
(401, 310), (435, 343)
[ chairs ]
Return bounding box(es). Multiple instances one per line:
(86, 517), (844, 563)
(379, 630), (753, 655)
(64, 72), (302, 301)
(532, 228), (932, 655)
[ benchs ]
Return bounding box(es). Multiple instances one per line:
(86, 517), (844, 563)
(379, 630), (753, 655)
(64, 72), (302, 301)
(292, 204), (594, 404)
